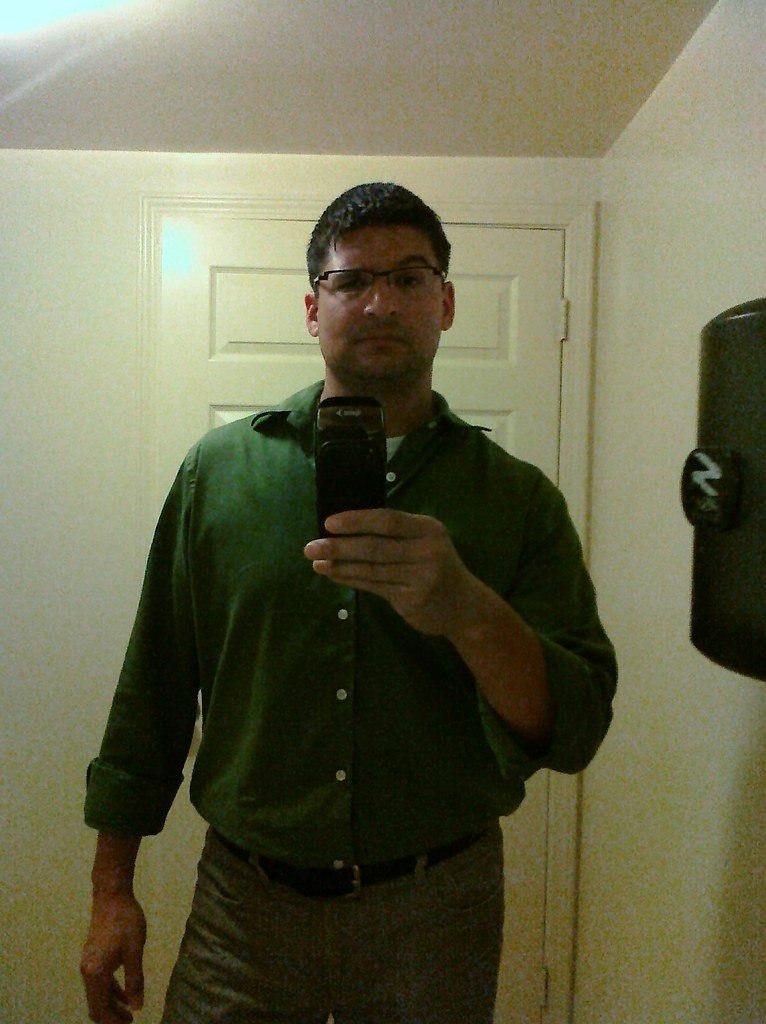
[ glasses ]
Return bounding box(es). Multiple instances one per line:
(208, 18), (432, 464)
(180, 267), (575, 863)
(312, 265), (446, 293)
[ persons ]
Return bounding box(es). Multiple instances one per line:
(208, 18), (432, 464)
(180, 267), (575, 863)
(76, 182), (620, 1024)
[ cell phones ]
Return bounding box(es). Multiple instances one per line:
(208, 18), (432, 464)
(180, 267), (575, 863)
(311, 393), (388, 536)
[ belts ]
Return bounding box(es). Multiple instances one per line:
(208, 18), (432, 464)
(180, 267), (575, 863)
(208, 826), (473, 899)
